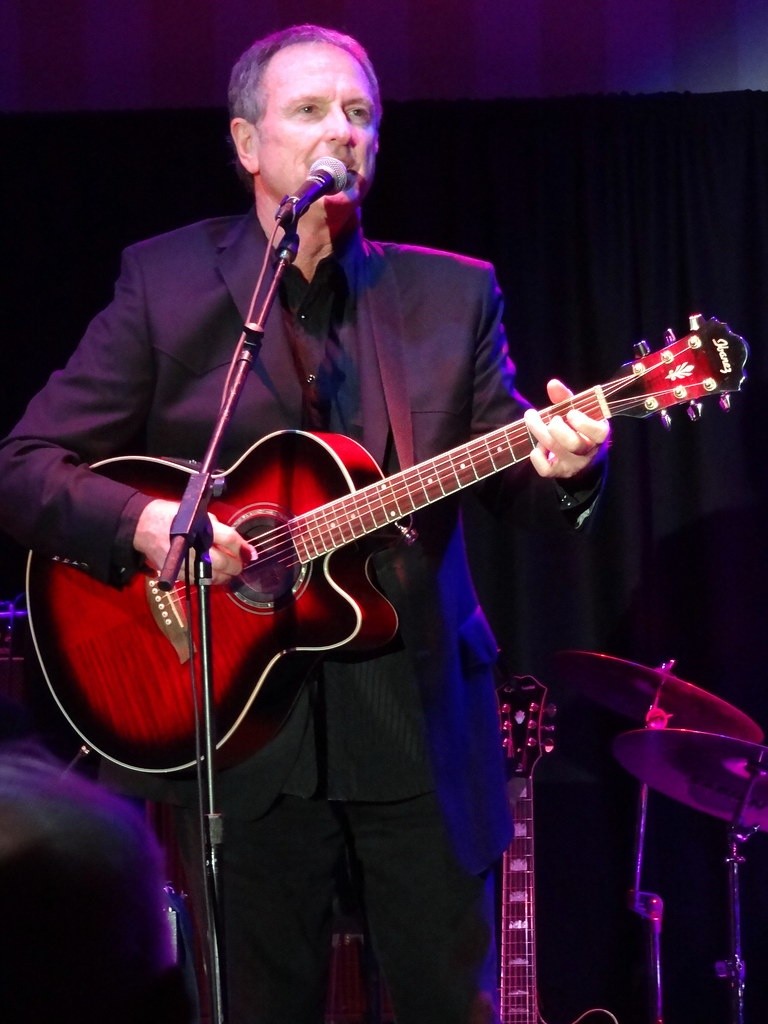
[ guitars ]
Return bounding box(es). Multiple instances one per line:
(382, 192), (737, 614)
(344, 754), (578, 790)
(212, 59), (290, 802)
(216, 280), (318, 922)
(467, 672), (620, 1024)
(25, 310), (752, 782)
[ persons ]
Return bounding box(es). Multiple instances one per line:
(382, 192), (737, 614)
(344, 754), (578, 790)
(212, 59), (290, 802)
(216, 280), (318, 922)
(0, 745), (199, 1024)
(0, 19), (611, 1024)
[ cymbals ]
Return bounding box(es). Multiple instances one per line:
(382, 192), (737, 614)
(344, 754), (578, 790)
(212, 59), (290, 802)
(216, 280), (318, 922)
(609, 727), (768, 833)
(551, 649), (766, 747)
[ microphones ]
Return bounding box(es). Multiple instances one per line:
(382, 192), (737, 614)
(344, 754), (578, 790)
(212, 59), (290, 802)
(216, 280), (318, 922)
(276, 156), (347, 222)
